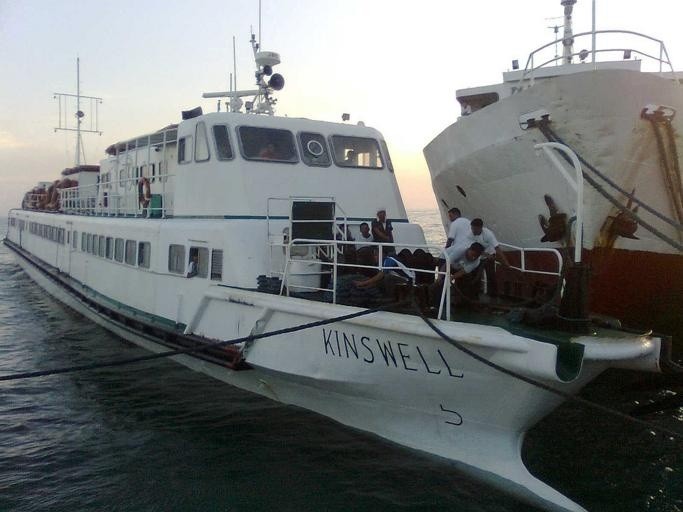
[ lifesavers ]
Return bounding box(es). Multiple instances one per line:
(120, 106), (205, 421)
(138, 178), (150, 208)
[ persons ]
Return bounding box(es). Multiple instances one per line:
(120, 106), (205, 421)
(184, 254), (199, 278)
(461, 102), (472, 116)
(259, 142), (282, 158)
(343, 150), (357, 166)
(335, 208), (512, 312)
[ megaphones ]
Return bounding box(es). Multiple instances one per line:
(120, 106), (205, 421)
(268, 73), (285, 91)
(255, 65), (272, 76)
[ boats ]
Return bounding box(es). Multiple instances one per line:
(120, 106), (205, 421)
(421, 1), (683, 338)
(0, 1), (674, 512)
